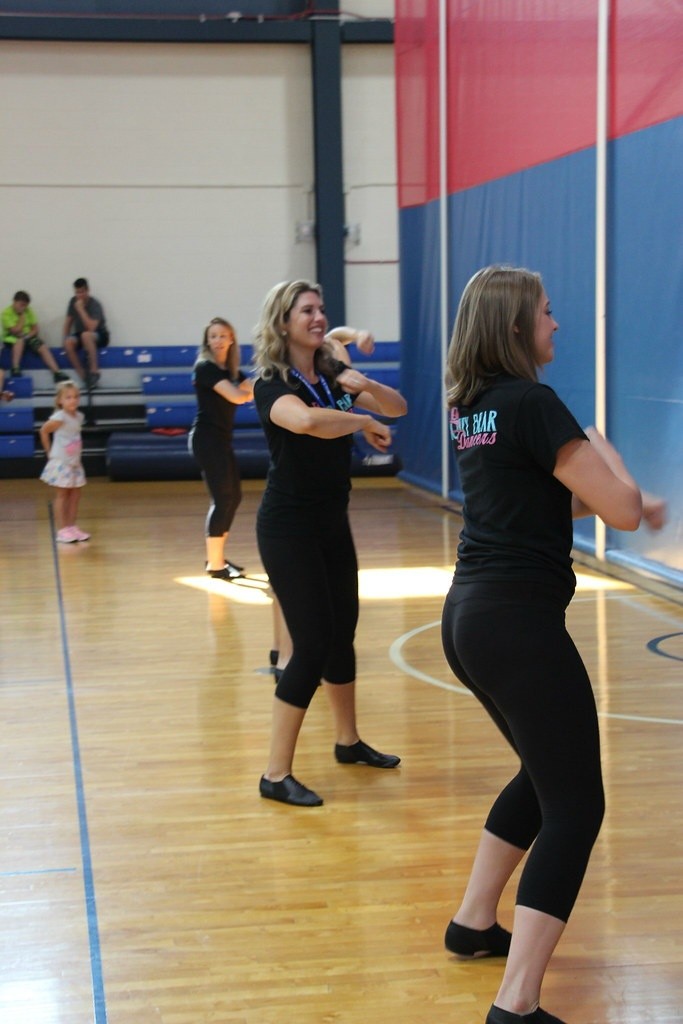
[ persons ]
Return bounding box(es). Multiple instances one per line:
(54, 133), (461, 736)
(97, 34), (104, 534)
(40, 381), (90, 543)
(188, 317), (257, 583)
(268, 326), (373, 683)
(62, 278), (109, 389)
(440, 268), (667, 1024)
(1, 290), (69, 382)
(254, 281), (407, 806)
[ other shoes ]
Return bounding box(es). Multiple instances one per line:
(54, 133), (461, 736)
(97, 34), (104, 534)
(207, 565), (244, 579)
(79, 372), (99, 393)
(485, 1004), (565, 1024)
(335, 739), (402, 769)
(270, 650), (279, 664)
(445, 916), (512, 957)
(53, 372), (70, 382)
(275, 668), (285, 683)
(206, 559), (243, 572)
(258, 771), (323, 806)
(11, 367), (22, 377)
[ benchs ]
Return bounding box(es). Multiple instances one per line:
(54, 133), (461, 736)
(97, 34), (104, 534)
(0, 335), (408, 480)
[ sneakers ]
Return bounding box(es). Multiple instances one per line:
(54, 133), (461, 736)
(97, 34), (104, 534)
(57, 525), (80, 543)
(70, 525), (91, 541)
